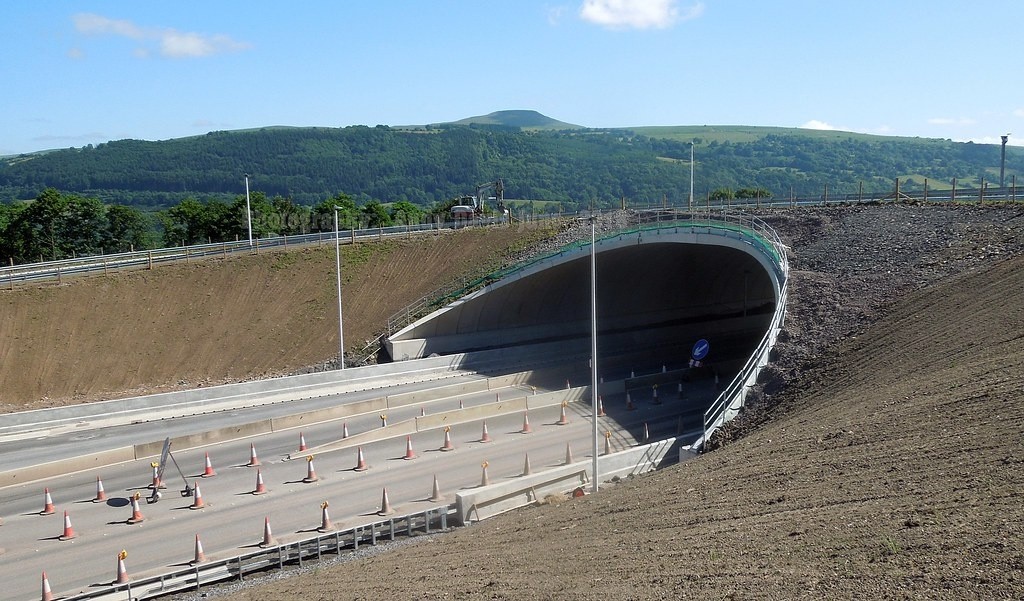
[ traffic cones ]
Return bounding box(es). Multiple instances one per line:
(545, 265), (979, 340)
(259, 515), (279, 548)
(353, 446), (369, 472)
(92, 475), (110, 503)
(252, 468), (269, 495)
(59, 509), (79, 541)
(342, 422), (351, 439)
(481, 462), (490, 486)
(201, 450), (218, 477)
(377, 487), (396, 516)
(298, 432), (310, 453)
(381, 362), (722, 467)
(39, 487), (59, 515)
(302, 455), (321, 483)
(147, 461), (168, 489)
(246, 443), (261, 467)
(427, 473), (446, 502)
(41, 571), (61, 601)
(520, 451), (534, 476)
(127, 494), (147, 524)
(317, 501), (337, 533)
(111, 552), (133, 587)
(189, 533), (213, 568)
(189, 482), (209, 509)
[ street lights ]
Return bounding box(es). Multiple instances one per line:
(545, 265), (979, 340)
(333, 204), (344, 364)
(244, 173), (252, 246)
(588, 214), (599, 493)
(1000, 135), (1008, 190)
(687, 141), (694, 208)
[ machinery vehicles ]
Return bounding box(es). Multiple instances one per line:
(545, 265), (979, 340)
(449, 177), (509, 222)
